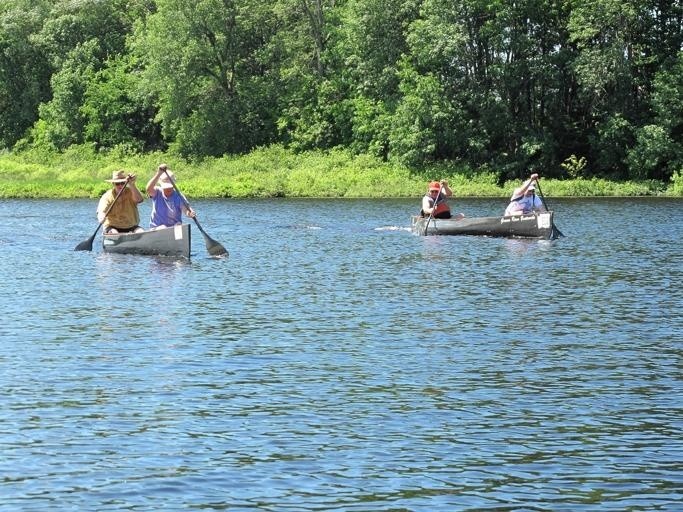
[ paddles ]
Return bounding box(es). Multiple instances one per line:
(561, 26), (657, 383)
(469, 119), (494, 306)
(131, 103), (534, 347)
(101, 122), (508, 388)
(162, 165), (228, 258)
(533, 177), (564, 241)
(420, 183), (444, 237)
(74, 173), (131, 252)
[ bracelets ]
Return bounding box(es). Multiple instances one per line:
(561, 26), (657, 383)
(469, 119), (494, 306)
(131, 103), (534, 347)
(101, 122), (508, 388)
(530, 178), (534, 182)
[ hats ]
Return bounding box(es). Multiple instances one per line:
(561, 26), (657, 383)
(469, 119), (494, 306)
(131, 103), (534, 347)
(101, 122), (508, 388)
(159, 170), (176, 189)
(428, 182), (440, 191)
(524, 179), (535, 189)
(104, 170), (128, 183)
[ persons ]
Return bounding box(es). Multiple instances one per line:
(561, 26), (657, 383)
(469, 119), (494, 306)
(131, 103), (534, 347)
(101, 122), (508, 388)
(146, 163), (196, 229)
(422, 179), (464, 220)
(505, 173), (544, 217)
(96, 170), (145, 234)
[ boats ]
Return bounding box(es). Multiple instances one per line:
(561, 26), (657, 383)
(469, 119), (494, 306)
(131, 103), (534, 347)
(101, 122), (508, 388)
(409, 209), (558, 242)
(100, 223), (193, 263)
